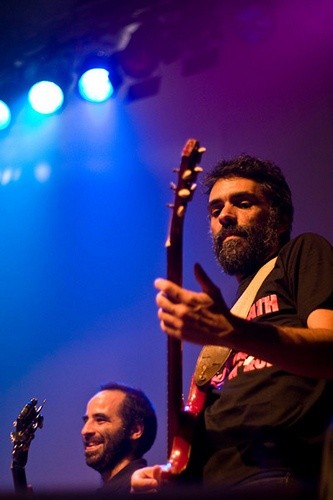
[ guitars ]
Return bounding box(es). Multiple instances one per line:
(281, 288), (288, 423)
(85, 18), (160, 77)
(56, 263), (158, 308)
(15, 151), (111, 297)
(157, 138), (213, 486)
(8, 397), (47, 496)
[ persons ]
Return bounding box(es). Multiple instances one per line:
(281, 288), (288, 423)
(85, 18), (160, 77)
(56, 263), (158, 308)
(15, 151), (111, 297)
(77, 381), (161, 499)
(126, 155), (332, 499)
